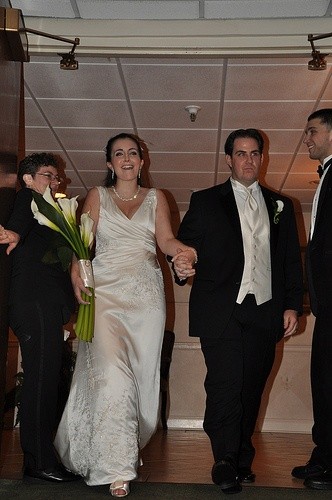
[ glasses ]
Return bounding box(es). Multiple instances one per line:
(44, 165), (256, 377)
(37, 172), (64, 184)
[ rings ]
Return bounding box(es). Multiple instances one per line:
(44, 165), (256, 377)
(296, 328), (298, 331)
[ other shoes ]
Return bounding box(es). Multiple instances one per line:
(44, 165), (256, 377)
(291, 463), (325, 478)
(236, 473), (256, 483)
(211, 461), (241, 495)
(303, 475), (332, 489)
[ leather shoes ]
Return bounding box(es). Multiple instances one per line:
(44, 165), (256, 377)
(24, 463), (82, 482)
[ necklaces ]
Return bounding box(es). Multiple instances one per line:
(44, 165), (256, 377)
(111, 184), (141, 202)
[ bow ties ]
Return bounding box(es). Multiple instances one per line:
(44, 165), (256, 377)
(317, 164), (325, 175)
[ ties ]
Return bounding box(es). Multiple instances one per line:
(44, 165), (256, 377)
(243, 187), (259, 231)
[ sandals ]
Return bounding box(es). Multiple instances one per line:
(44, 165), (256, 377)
(110, 479), (130, 498)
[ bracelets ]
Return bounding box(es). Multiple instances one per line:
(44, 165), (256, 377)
(188, 248), (198, 264)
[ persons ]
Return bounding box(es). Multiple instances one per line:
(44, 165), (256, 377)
(0, 152), (76, 483)
(166, 128), (305, 495)
(53, 132), (199, 497)
(290, 108), (332, 491)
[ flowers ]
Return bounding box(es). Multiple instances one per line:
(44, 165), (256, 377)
(30, 182), (97, 344)
(273, 199), (285, 226)
